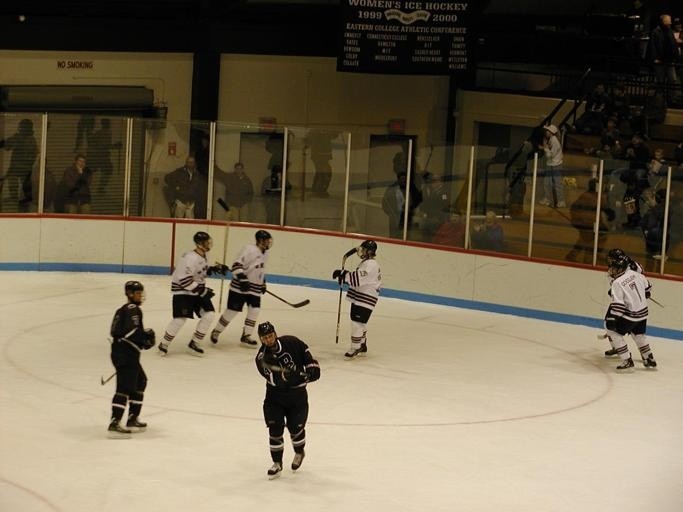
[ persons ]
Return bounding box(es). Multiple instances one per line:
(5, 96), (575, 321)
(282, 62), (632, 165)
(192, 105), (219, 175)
(603, 248), (645, 356)
(650, 14), (683, 109)
(432, 208), (466, 248)
(418, 171), (435, 243)
(214, 161), (254, 223)
(538, 124), (566, 208)
(94, 118), (123, 193)
(332, 239), (384, 358)
(163, 155), (203, 220)
(0, 118), (40, 214)
(72, 113), (96, 152)
(260, 164), (292, 226)
(107, 280), (156, 435)
(158, 231), (229, 355)
(61, 153), (93, 215)
(393, 136), (423, 231)
(265, 128), (290, 190)
(461, 211), (505, 253)
(565, 83), (673, 274)
(209, 229), (274, 346)
(382, 170), (422, 240)
(29, 153), (56, 214)
(429, 172), (452, 244)
(254, 321), (321, 475)
(303, 128), (340, 198)
(605, 257), (657, 370)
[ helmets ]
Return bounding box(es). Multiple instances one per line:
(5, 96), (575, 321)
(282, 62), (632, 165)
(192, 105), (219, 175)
(258, 322), (278, 349)
(124, 280), (146, 305)
(255, 230), (272, 250)
(193, 232), (212, 252)
(606, 248), (624, 266)
(611, 258), (627, 278)
(357, 239), (377, 258)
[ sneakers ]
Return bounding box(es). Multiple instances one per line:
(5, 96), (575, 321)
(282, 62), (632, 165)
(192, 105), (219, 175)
(108, 420), (131, 434)
(344, 346), (363, 357)
(211, 329), (218, 343)
(291, 451), (305, 470)
(188, 340), (203, 353)
(126, 416), (147, 427)
(617, 357), (634, 369)
(604, 348), (618, 357)
(158, 343), (167, 353)
(643, 354), (656, 368)
(240, 335), (257, 345)
(363, 344), (368, 353)
(268, 460), (283, 476)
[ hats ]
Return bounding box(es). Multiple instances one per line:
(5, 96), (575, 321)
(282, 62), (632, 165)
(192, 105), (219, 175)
(544, 125), (558, 134)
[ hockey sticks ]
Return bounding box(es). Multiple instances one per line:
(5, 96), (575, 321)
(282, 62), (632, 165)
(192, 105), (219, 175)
(335, 247), (360, 345)
(215, 261), (311, 309)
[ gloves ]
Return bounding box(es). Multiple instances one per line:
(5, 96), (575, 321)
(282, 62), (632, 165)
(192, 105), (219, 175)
(210, 264), (228, 275)
(306, 362), (320, 383)
(605, 318), (616, 332)
(238, 274), (249, 292)
(198, 288), (215, 301)
(332, 270), (349, 278)
